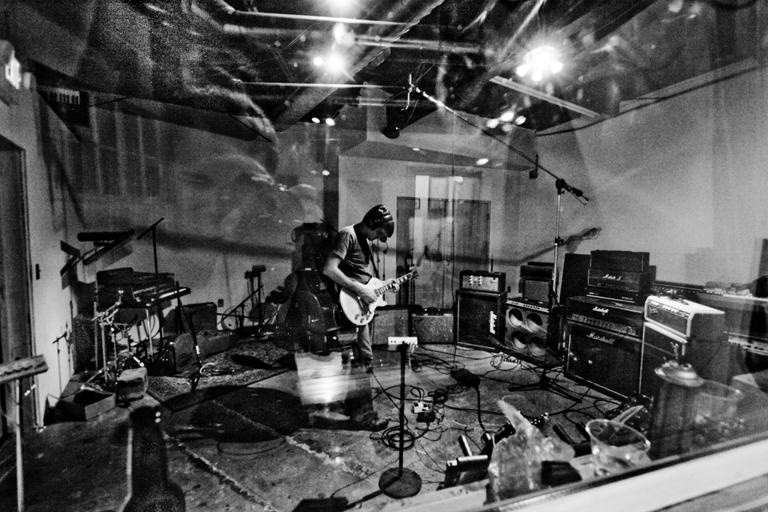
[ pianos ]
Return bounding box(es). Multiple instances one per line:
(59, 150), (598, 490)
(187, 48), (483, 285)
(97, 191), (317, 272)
(95, 266), (192, 309)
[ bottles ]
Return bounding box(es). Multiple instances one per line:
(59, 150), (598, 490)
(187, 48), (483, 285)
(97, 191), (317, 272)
(649, 358), (703, 443)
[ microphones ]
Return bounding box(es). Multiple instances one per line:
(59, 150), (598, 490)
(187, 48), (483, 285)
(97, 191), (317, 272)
(136, 218), (164, 239)
(403, 72), (413, 111)
(380, 237), (390, 254)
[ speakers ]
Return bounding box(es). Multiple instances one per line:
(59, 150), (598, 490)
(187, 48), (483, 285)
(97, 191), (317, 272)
(504, 298), (561, 367)
(524, 280), (550, 300)
(587, 250), (649, 293)
(455, 289), (501, 352)
(562, 321), (641, 401)
(639, 323), (729, 403)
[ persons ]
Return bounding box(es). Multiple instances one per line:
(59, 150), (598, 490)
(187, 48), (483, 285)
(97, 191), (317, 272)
(91, 83), (322, 320)
(322, 206), (400, 431)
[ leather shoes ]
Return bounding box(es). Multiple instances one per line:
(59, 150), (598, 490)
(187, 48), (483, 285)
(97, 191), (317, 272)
(348, 417), (388, 431)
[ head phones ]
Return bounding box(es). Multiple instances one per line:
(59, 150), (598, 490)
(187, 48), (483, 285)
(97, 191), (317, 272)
(366, 214), (393, 229)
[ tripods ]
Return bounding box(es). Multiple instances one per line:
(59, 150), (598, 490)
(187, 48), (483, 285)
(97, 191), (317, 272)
(83, 327), (115, 385)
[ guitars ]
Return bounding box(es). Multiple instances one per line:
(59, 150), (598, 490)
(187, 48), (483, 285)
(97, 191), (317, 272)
(337, 264), (419, 327)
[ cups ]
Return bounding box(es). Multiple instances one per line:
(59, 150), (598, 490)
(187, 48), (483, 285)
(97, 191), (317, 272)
(691, 379), (743, 436)
(581, 418), (652, 479)
